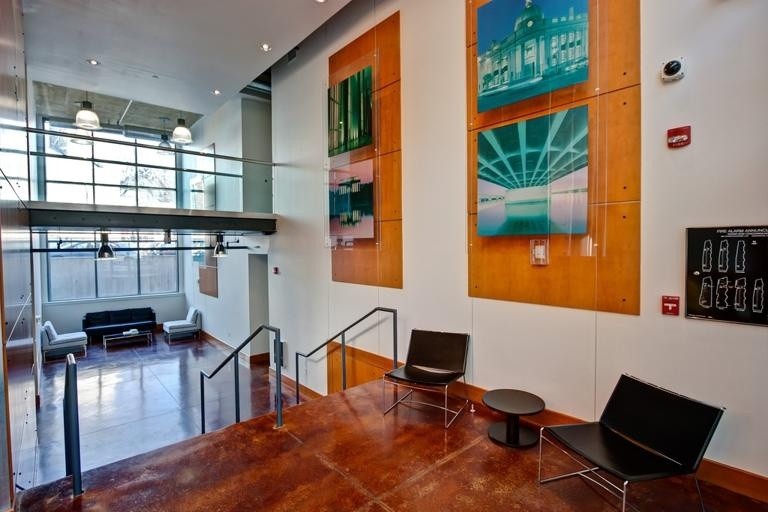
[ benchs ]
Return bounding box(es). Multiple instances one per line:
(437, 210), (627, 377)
(538, 371), (714, 512)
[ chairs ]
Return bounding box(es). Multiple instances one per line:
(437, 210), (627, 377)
(380, 326), (470, 425)
(161, 307), (202, 345)
(43, 320), (90, 359)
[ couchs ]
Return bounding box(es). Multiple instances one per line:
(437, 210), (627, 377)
(81, 306), (157, 341)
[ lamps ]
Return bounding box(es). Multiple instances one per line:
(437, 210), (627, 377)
(96, 233), (115, 260)
(212, 233), (229, 258)
(156, 108), (193, 157)
(75, 88), (101, 129)
